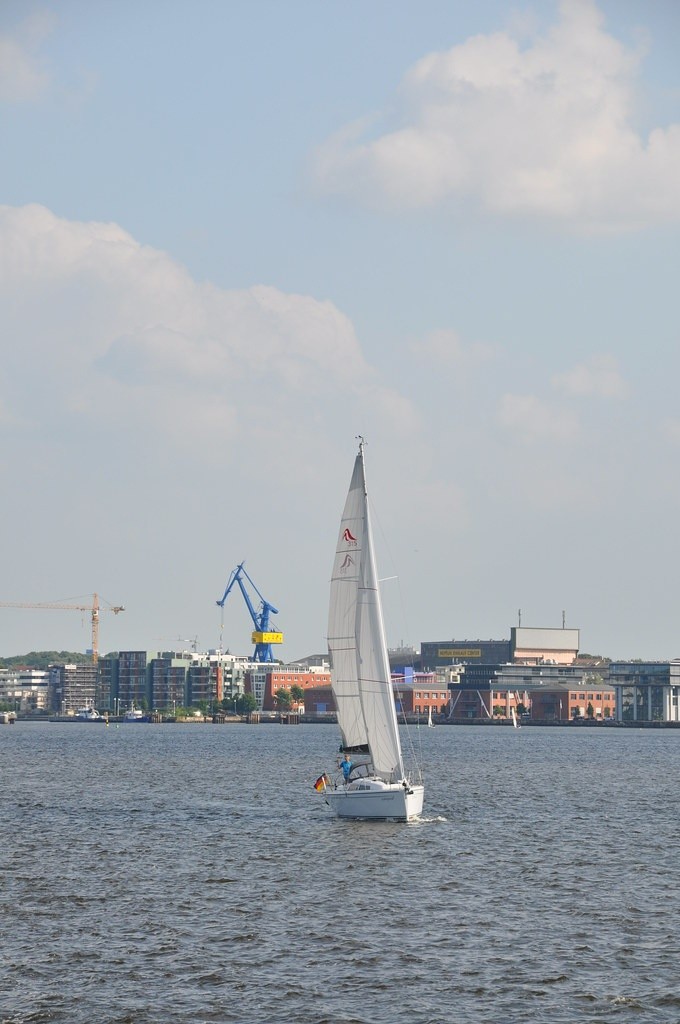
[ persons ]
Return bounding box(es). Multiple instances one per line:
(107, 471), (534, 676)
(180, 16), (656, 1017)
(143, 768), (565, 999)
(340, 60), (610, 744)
(337, 754), (354, 785)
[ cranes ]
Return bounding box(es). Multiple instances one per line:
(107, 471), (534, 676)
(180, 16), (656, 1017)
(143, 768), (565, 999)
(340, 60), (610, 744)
(1, 593), (127, 669)
(214, 560), (285, 663)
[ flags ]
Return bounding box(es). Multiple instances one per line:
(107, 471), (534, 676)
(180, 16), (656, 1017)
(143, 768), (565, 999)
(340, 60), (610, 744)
(317, 773), (329, 786)
(314, 777), (324, 792)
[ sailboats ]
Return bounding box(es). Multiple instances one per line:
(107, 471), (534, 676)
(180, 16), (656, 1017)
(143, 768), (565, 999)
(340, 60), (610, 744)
(314, 439), (428, 823)
